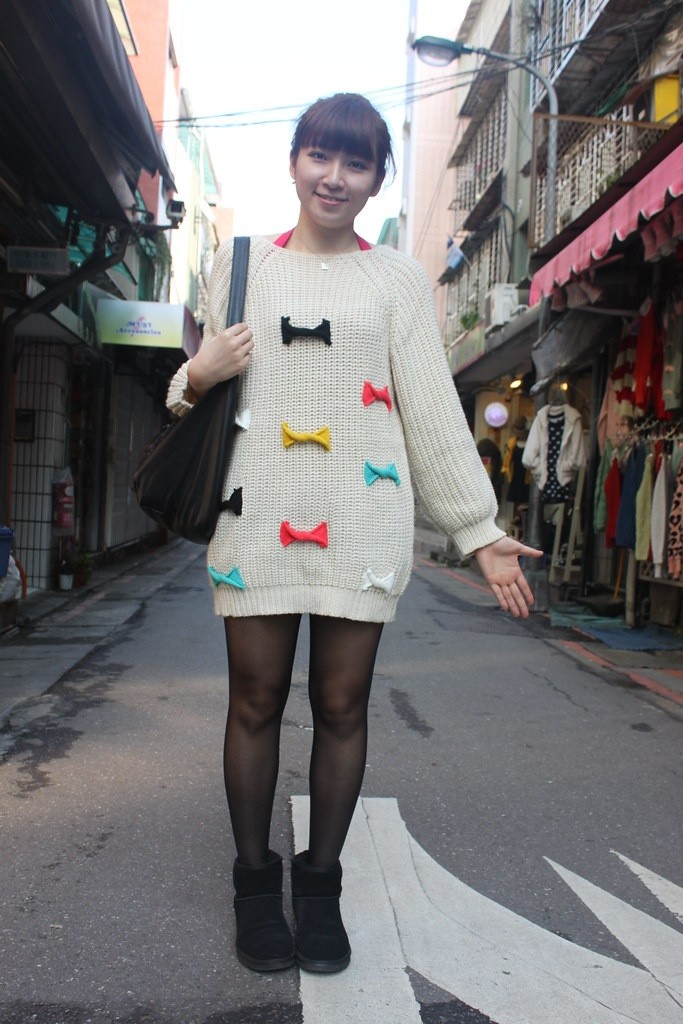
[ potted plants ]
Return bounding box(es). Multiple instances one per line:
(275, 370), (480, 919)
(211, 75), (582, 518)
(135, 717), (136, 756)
(57, 554), (75, 590)
(76, 554), (91, 585)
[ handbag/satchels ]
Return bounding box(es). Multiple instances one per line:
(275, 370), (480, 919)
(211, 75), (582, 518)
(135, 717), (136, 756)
(132, 233), (251, 541)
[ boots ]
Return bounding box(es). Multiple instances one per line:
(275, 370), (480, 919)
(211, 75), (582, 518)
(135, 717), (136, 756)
(290, 850), (350, 975)
(232, 851), (297, 970)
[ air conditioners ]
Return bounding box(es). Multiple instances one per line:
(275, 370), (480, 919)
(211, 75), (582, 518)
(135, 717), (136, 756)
(481, 281), (519, 334)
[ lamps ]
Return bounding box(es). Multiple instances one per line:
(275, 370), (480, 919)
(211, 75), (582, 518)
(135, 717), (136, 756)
(551, 269), (606, 313)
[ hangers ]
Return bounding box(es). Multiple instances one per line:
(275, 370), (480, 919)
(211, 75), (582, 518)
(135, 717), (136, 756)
(547, 392), (574, 409)
(613, 415), (681, 445)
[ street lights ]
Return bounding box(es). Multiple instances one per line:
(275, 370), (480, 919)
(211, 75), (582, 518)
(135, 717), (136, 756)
(414, 34), (558, 566)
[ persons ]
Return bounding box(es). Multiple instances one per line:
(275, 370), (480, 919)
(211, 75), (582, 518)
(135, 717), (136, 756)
(501, 416), (531, 503)
(166, 94), (544, 975)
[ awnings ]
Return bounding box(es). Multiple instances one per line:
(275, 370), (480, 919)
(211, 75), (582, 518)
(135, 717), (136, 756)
(445, 296), (554, 384)
(527, 112), (683, 304)
